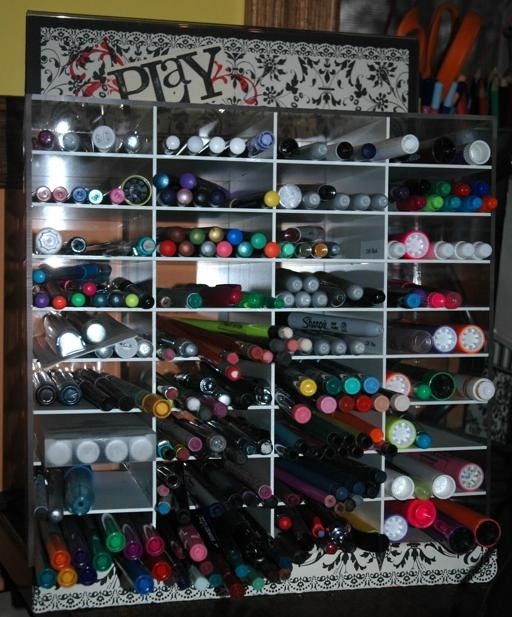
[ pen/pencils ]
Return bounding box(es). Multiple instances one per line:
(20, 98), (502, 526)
(421, 66), (509, 118)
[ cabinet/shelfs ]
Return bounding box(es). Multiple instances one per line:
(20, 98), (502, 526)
(2, 91), (512, 615)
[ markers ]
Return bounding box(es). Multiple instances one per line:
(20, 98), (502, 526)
(24, 124), (502, 598)
